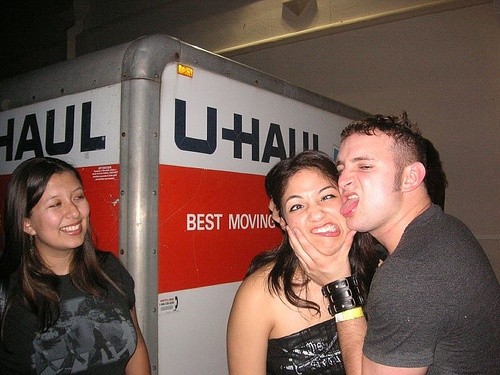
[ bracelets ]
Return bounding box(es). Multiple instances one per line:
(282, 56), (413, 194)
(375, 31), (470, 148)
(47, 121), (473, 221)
(318, 274), (371, 326)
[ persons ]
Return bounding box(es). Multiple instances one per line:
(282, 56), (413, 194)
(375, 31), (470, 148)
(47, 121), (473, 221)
(0, 156), (154, 375)
(268, 116), (500, 375)
(224, 151), (398, 375)
